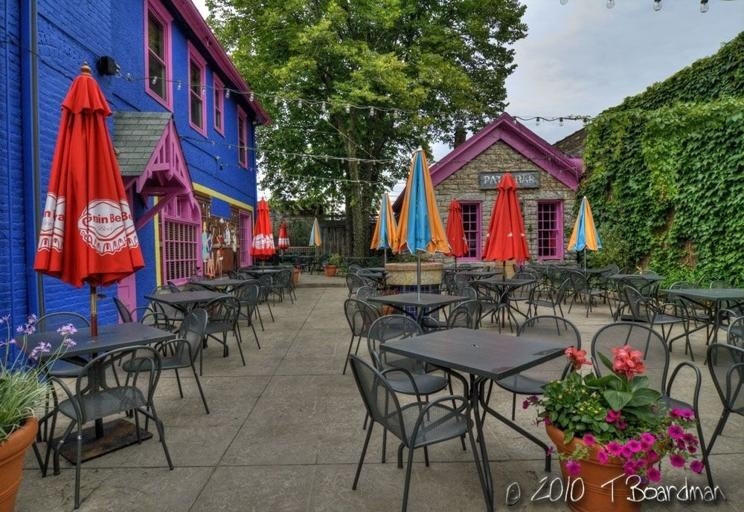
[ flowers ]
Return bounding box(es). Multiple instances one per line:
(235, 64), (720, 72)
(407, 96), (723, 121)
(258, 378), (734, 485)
(0, 310), (78, 446)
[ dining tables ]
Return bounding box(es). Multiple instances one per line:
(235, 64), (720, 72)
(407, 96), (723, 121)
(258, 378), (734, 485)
(326, 252), (342, 277)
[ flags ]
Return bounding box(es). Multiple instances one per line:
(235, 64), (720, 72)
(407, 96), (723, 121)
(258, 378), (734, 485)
(0, 416), (38, 511)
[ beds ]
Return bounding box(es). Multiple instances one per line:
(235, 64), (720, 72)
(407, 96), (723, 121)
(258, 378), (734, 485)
(278, 220), (290, 262)
(308, 217), (323, 256)
(481, 170), (532, 283)
(566, 196), (603, 268)
(369, 191), (399, 265)
(250, 196), (276, 272)
(32, 62), (145, 336)
(392, 145), (450, 293)
(442, 197), (470, 262)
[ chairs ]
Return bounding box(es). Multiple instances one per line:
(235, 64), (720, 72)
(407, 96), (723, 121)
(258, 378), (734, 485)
(14, 265), (297, 508)
(341, 260), (744, 512)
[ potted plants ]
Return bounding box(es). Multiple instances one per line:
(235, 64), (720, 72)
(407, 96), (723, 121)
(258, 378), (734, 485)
(326, 252), (342, 277)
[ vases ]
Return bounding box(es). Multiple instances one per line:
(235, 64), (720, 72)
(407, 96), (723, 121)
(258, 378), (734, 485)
(0, 310), (78, 446)
(0, 416), (38, 511)
